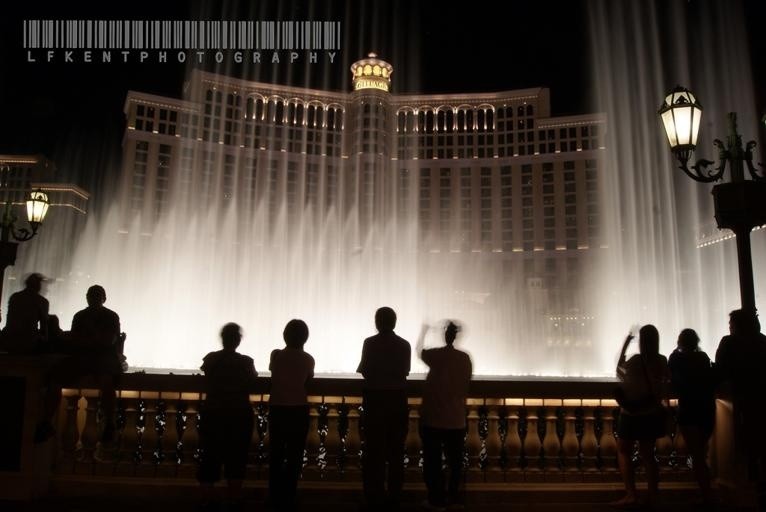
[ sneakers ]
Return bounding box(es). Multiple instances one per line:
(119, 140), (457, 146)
(34, 423), (55, 443)
(422, 498), (447, 511)
(199, 497), (241, 511)
(448, 502), (465, 511)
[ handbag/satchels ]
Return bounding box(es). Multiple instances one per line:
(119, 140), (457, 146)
(612, 384), (656, 413)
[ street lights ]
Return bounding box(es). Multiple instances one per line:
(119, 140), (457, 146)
(0, 187), (48, 320)
(658, 89), (765, 493)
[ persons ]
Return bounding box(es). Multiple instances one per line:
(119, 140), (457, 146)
(3, 273), (50, 357)
(195, 322), (259, 510)
(714, 310), (766, 510)
(609, 322), (669, 510)
(266, 319), (316, 512)
(416, 316), (472, 510)
(671, 329), (719, 509)
(67, 284), (121, 376)
(355, 306), (412, 510)
